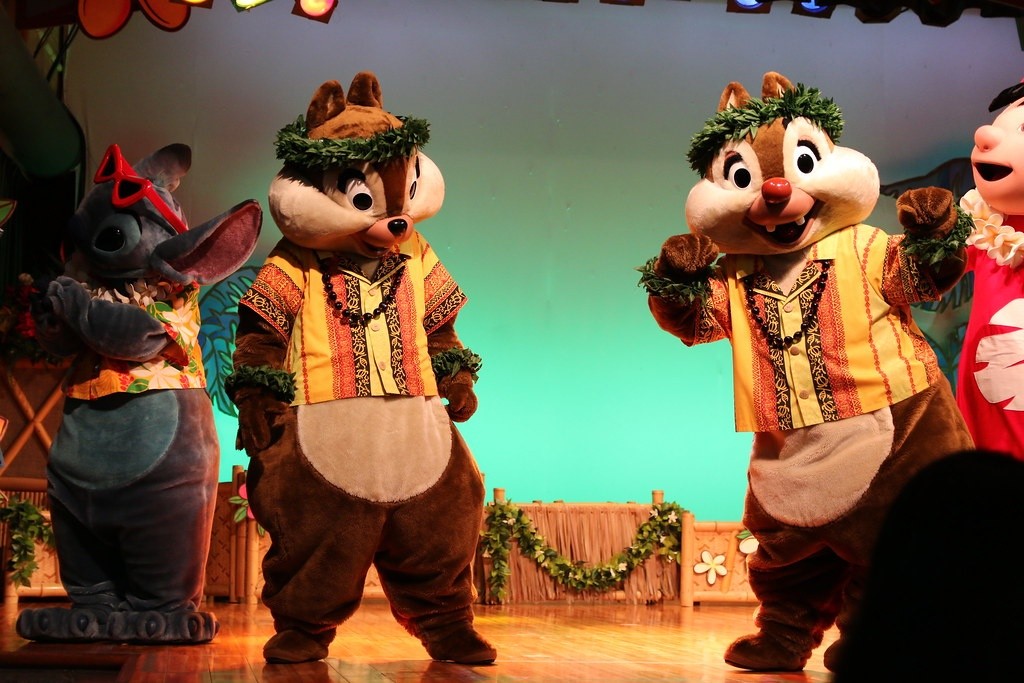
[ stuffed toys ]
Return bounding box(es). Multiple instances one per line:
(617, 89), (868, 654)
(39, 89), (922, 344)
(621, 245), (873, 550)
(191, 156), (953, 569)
(223, 70), (497, 664)
(955, 75), (1024, 468)
(633, 71), (978, 673)
(16, 142), (263, 645)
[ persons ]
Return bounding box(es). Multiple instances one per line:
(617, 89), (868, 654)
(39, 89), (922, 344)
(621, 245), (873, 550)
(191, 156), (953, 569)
(827, 447), (1024, 683)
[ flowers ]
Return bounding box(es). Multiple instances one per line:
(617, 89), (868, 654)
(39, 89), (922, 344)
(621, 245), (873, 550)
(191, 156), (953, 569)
(694, 550), (728, 585)
(479, 497), (682, 602)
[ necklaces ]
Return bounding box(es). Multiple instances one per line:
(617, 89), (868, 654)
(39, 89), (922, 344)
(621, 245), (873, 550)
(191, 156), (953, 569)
(319, 252), (404, 321)
(961, 184), (1024, 269)
(745, 260), (832, 346)
